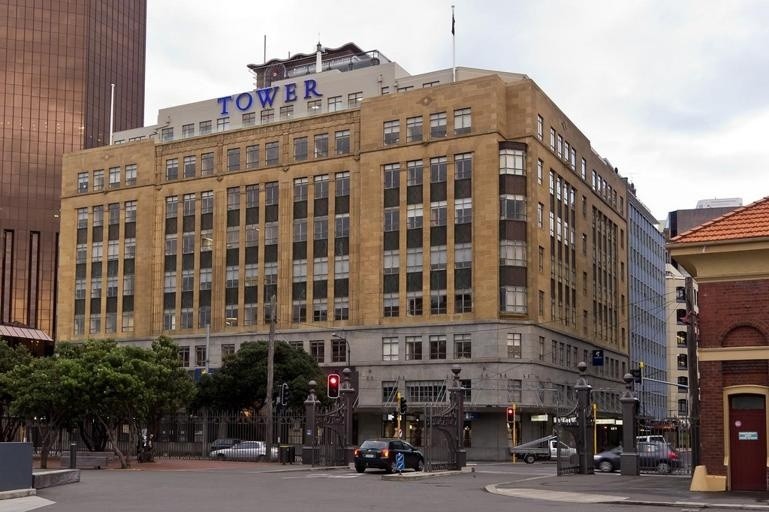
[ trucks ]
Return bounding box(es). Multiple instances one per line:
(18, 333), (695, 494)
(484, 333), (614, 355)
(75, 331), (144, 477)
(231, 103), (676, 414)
(508, 434), (577, 466)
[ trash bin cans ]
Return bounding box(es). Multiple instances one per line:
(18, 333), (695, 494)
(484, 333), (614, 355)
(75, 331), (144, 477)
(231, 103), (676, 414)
(456, 451), (466, 467)
(280, 446), (295, 462)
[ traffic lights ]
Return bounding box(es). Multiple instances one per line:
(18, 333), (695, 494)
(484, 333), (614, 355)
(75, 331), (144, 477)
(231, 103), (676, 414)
(392, 410), (400, 429)
(281, 383), (289, 407)
(399, 396), (406, 415)
(629, 361), (644, 385)
(506, 406), (515, 424)
(327, 373), (341, 400)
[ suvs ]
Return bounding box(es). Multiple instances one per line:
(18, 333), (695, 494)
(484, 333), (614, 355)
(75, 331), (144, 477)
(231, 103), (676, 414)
(208, 436), (243, 452)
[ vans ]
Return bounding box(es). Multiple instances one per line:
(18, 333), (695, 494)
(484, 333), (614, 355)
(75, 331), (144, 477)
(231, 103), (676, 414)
(635, 434), (667, 446)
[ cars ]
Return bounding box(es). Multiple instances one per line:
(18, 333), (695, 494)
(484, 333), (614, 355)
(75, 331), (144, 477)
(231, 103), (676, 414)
(676, 447), (692, 475)
(354, 437), (426, 473)
(593, 441), (683, 475)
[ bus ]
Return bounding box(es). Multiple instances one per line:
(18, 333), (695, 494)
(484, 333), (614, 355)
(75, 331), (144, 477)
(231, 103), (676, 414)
(209, 440), (278, 462)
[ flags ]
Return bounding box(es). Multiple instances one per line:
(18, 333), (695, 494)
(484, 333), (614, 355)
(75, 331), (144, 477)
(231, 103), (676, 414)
(452, 12), (455, 36)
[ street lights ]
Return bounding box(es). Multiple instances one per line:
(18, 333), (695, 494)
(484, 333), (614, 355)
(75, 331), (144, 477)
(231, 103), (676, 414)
(331, 332), (352, 368)
